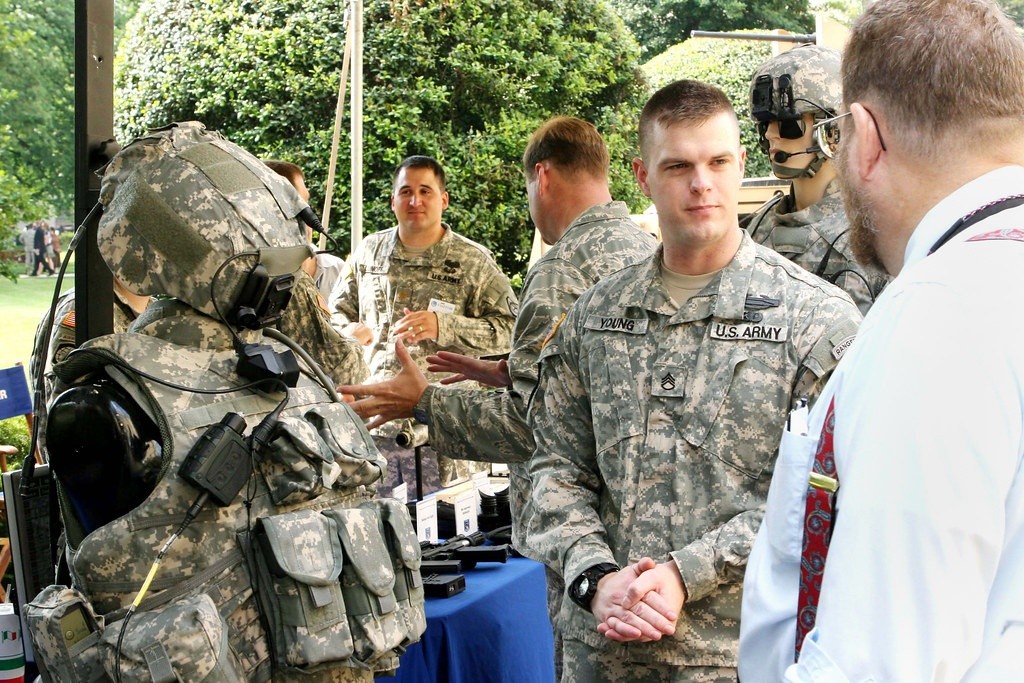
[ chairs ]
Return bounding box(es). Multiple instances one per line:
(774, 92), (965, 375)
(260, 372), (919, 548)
(0, 464), (71, 683)
(0, 362), (44, 474)
(0, 445), (18, 601)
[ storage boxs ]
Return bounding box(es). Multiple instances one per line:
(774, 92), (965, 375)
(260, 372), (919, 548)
(408, 498), (437, 542)
(437, 495), (479, 539)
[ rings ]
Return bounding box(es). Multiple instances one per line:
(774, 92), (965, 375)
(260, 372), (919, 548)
(419, 326), (423, 332)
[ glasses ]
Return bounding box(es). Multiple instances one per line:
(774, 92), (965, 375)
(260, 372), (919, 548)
(811, 107), (886, 160)
(757, 118), (807, 141)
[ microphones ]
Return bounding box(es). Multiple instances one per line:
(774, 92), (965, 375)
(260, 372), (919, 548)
(774, 144), (820, 163)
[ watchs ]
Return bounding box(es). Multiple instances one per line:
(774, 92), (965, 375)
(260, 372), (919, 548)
(574, 564), (620, 611)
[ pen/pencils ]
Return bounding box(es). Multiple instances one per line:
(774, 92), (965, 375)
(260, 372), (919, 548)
(786, 398), (809, 435)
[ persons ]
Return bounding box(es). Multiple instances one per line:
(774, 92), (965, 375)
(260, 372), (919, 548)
(24, 122), (429, 683)
(526, 79), (866, 683)
(743, 44), (888, 314)
(335, 118), (662, 683)
(737, 0), (1024, 683)
(18, 124), (517, 683)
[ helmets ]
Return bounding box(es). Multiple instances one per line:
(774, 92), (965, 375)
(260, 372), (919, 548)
(749, 45), (842, 121)
(98, 120), (314, 329)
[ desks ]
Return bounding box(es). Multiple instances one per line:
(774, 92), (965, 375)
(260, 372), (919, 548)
(373, 534), (557, 683)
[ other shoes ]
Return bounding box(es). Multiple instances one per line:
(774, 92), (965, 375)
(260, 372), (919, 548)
(48, 270), (55, 275)
(29, 273), (37, 275)
(33, 273), (34, 274)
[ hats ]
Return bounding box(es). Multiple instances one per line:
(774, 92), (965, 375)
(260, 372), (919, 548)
(26, 222), (32, 226)
